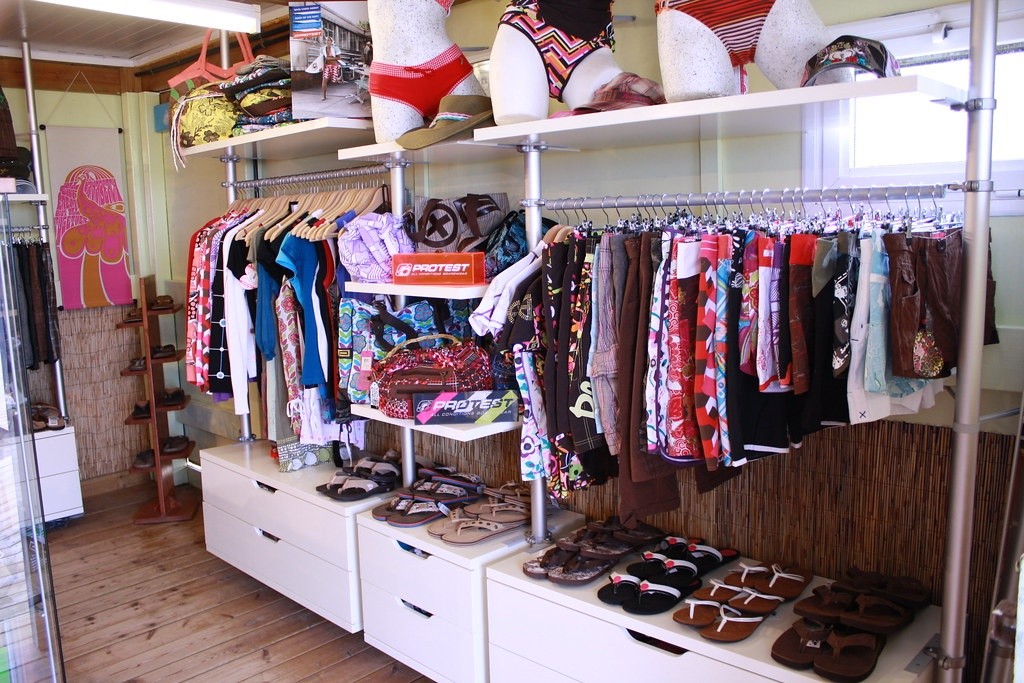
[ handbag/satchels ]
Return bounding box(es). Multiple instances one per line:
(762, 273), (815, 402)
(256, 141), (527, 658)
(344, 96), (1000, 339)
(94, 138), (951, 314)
(408, 186), (559, 283)
(377, 329), (499, 419)
(164, 23), (292, 172)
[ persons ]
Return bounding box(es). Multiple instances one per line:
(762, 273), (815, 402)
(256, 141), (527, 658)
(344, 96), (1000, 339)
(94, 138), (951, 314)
(362, 39), (374, 68)
(491, 0), (623, 129)
(320, 36), (347, 101)
(655, 1), (850, 106)
(365, 0), (491, 148)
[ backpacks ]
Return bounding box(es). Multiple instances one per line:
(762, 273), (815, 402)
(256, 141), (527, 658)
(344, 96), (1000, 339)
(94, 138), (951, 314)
(334, 207), (420, 285)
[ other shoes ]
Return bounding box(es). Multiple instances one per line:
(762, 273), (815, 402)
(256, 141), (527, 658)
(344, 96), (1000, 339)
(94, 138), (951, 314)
(159, 389), (185, 406)
(132, 401), (152, 419)
(136, 449), (156, 466)
(128, 356), (148, 371)
(151, 344), (176, 359)
(160, 435), (191, 454)
(122, 307), (143, 323)
(146, 295), (175, 311)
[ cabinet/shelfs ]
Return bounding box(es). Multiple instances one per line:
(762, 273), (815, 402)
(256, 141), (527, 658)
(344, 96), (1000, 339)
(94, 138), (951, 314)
(115, 273), (202, 526)
(178, 114), (403, 635)
(334, 141), (586, 682)
(0, 190), (88, 527)
(469, 70), (997, 682)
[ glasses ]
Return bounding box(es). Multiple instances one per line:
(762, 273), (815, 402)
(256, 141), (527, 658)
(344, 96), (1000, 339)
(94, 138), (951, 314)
(798, 32), (902, 85)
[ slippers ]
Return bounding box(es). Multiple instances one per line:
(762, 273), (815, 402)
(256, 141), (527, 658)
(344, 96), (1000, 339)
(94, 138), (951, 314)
(315, 447), (532, 547)
(521, 515), (934, 681)
(28, 402), (66, 432)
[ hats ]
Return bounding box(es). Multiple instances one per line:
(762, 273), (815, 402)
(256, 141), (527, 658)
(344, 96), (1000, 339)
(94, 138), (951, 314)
(397, 92), (494, 153)
(576, 72), (668, 114)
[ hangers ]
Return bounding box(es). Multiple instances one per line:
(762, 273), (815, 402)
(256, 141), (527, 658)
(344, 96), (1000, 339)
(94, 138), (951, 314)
(542, 182), (964, 246)
(221, 165), (410, 246)
